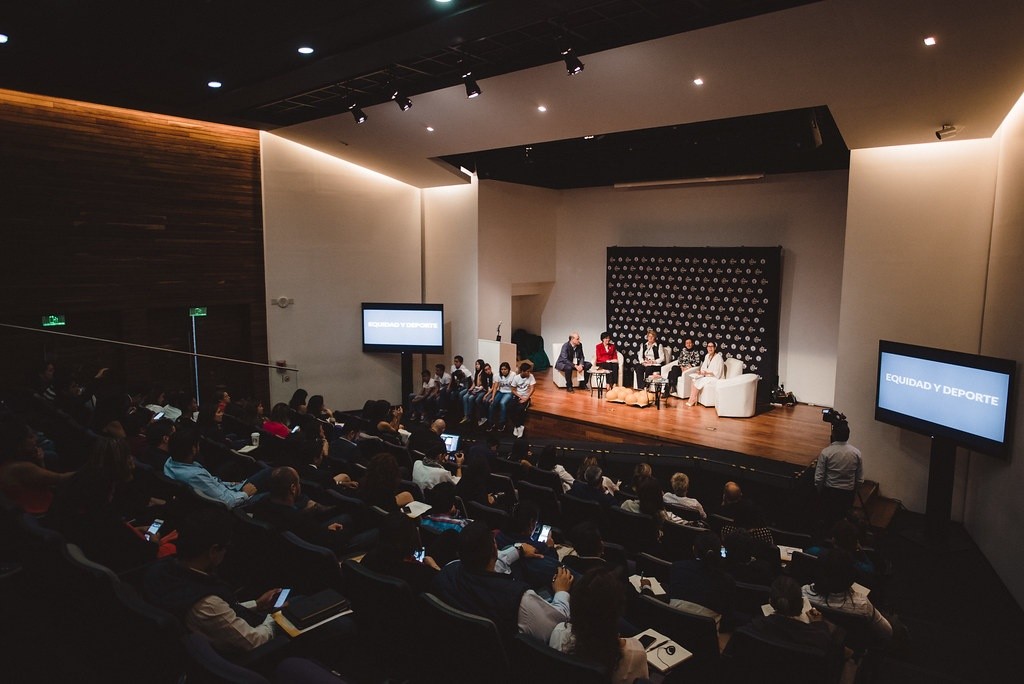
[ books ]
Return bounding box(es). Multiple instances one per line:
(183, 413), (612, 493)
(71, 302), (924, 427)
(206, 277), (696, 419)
(633, 628), (693, 672)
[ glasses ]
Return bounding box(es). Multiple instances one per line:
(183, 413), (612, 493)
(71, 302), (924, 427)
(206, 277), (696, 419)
(707, 346), (713, 348)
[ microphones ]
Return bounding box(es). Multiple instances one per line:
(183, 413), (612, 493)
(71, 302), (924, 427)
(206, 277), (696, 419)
(497, 321), (503, 331)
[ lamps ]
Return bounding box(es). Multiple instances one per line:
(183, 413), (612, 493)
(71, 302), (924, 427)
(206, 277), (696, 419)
(935, 124), (956, 140)
(558, 39), (584, 76)
(385, 85), (412, 112)
(808, 111), (823, 149)
(344, 94), (368, 122)
(458, 60), (482, 99)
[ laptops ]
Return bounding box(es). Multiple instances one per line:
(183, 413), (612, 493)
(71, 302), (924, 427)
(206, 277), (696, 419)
(440, 433), (459, 461)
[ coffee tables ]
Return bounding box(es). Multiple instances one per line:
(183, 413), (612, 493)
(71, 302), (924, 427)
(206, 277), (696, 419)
(643, 378), (669, 409)
(587, 369), (613, 399)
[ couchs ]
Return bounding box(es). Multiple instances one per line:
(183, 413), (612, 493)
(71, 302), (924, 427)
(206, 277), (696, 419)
(589, 350), (624, 389)
(695, 358), (744, 407)
(659, 360), (704, 398)
(715, 372), (759, 417)
(634, 348), (673, 392)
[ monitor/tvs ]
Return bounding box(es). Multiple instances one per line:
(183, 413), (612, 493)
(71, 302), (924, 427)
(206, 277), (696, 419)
(875, 340), (1016, 465)
(362, 302), (444, 354)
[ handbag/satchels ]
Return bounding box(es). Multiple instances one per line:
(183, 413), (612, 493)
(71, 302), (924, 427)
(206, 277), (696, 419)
(689, 372), (704, 379)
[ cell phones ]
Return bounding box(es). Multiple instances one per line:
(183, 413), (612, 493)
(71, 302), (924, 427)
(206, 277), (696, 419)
(402, 507), (411, 514)
(720, 547), (726, 558)
(414, 546), (425, 563)
(291, 426), (300, 433)
(143, 519), (164, 542)
(273, 588), (290, 608)
(638, 635), (657, 649)
(153, 411), (165, 420)
(538, 524), (551, 543)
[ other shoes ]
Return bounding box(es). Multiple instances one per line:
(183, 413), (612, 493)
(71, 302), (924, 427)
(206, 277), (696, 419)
(580, 383), (590, 390)
(567, 386), (573, 391)
(662, 391), (670, 398)
(671, 387), (678, 394)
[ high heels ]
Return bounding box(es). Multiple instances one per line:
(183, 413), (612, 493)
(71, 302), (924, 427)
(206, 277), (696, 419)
(686, 401), (690, 405)
(688, 397), (697, 407)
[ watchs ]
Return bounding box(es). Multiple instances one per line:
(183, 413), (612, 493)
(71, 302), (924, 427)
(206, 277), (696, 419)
(514, 542), (523, 556)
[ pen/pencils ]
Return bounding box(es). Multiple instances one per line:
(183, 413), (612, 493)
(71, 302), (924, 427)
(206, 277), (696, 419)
(647, 640), (669, 653)
(553, 565), (565, 581)
(641, 571), (644, 579)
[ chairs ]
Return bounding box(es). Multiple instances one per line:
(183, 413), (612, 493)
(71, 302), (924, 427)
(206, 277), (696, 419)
(0, 395), (885, 684)
(552, 343), (581, 389)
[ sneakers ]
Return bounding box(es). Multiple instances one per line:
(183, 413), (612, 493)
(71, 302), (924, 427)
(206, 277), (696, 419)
(498, 424), (505, 431)
(513, 427), (519, 435)
(420, 416), (427, 423)
(479, 417), (487, 425)
(516, 425), (524, 439)
(486, 422), (495, 432)
(410, 414), (418, 420)
(459, 418), (470, 424)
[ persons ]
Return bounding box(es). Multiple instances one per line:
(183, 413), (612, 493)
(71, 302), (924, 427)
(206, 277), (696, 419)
(595, 332), (619, 390)
(0, 356), (893, 684)
(555, 333), (592, 393)
(636, 330), (666, 389)
(685, 341), (725, 406)
(661, 337), (701, 398)
